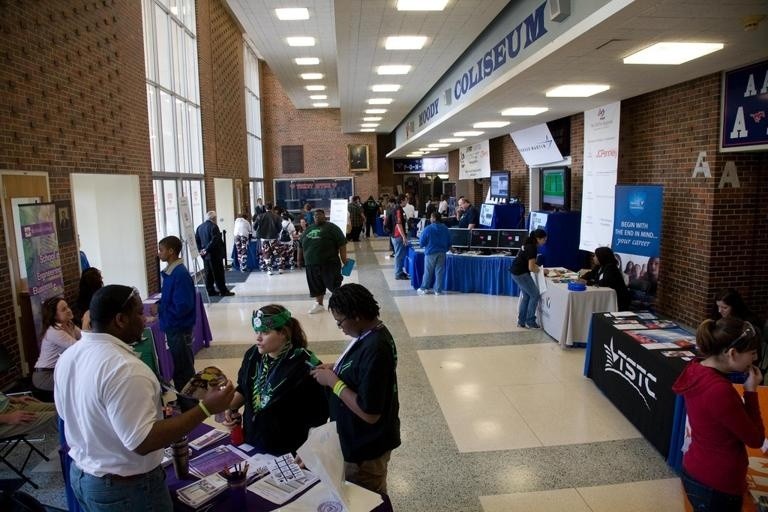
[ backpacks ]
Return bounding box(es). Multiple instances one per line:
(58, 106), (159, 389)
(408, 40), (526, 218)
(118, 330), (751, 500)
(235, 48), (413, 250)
(280, 223), (291, 242)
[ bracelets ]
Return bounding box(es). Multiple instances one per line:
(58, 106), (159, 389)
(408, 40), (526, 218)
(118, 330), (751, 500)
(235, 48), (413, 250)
(198, 399), (212, 418)
(332, 379), (347, 397)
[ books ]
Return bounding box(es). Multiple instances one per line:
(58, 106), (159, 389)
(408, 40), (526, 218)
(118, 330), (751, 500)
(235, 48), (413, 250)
(175, 428), (320, 512)
(602, 310), (697, 357)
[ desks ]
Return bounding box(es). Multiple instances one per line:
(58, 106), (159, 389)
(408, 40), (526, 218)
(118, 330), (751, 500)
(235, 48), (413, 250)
(231, 242), (258, 271)
(58, 291), (394, 512)
(406, 245), (543, 299)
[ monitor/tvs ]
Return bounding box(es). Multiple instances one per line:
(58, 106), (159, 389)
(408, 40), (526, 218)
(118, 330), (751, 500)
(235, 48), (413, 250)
(539, 165), (571, 211)
(490, 170), (511, 204)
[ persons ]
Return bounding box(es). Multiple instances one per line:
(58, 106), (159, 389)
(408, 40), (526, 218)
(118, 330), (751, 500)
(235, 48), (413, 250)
(233, 198), (314, 274)
(672, 316), (765, 511)
(223, 304), (329, 468)
(0, 267), (103, 445)
(311, 284), (401, 493)
(508, 229), (548, 329)
(297, 210), (348, 314)
(54, 285), (235, 512)
(149, 236), (196, 409)
(614, 253), (660, 297)
(575, 247), (632, 311)
(347, 191), (480, 295)
(714, 289), (763, 366)
(195, 211), (235, 296)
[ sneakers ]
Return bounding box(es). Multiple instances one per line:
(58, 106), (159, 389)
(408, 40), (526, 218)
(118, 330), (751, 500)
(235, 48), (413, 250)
(308, 302), (325, 314)
(397, 270), (409, 280)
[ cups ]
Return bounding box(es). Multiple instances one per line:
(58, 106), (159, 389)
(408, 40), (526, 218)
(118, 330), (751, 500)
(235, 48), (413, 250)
(228, 471), (246, 511)
(171, 436), (189, 478)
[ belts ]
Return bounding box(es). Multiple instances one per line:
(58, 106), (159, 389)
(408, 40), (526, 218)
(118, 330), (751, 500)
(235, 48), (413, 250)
(32, 368), (55, 372)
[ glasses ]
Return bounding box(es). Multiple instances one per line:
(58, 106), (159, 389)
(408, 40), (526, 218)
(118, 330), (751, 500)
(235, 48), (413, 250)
(724, 320), (756, 354)
(123, 285), (140, 308)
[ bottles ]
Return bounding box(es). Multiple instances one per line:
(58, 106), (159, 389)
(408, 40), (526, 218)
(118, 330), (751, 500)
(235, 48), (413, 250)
(230, 407), (243, 444)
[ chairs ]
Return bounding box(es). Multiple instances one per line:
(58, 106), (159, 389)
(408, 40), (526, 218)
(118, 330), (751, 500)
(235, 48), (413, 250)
(0, 434), (49, 489)
(0, 344), (54, 444)
(580, 310), (766, 472)
(517, 266), (621, 352)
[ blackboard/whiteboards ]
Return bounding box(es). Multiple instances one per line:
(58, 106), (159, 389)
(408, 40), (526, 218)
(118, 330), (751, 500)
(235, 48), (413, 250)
(273, 177), (354, 213)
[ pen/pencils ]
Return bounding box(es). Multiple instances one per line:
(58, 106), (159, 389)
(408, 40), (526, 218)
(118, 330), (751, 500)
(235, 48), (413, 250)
(223, 462), (249, 478)
(305, 361), (316, 370)
